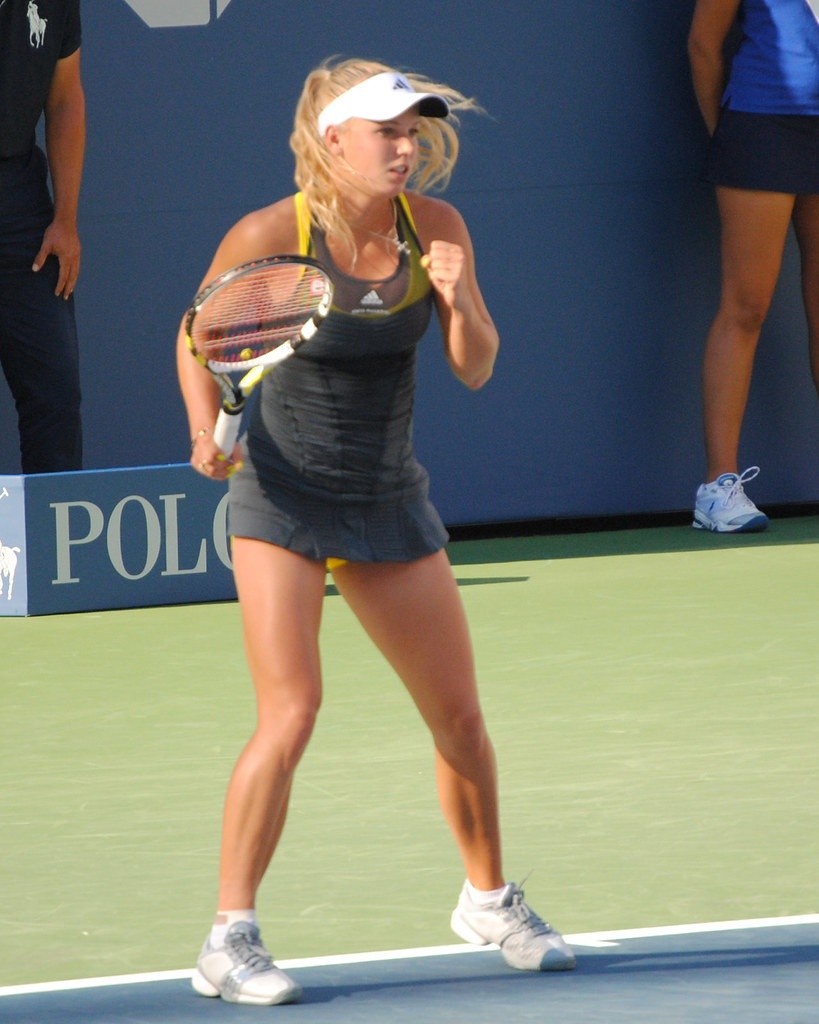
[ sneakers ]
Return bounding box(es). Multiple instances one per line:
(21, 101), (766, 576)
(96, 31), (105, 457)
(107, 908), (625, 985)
(450, 877), (577, 970)
(191, 920), (304, 1004)
(691, 482), (771, 534)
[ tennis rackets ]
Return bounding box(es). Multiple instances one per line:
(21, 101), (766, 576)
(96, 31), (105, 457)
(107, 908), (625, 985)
(182, 252), (337, 478)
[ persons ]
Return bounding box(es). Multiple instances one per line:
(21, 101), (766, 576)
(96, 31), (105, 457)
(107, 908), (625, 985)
(175, 53), (577, 1007)
(0, 0), (86, 475)
(686, 0), (819, 534)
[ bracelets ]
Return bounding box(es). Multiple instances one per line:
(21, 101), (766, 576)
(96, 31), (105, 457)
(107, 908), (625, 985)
(191, 426), (211, 449)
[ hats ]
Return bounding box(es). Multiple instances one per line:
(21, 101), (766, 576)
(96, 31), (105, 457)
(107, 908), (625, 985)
(315, 68), (452, 136)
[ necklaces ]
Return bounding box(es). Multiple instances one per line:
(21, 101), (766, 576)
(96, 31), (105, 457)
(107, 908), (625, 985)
(349, 223), (411, 255)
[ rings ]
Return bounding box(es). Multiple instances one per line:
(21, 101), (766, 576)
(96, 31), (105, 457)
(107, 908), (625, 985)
(198, 458), (209, 469)
